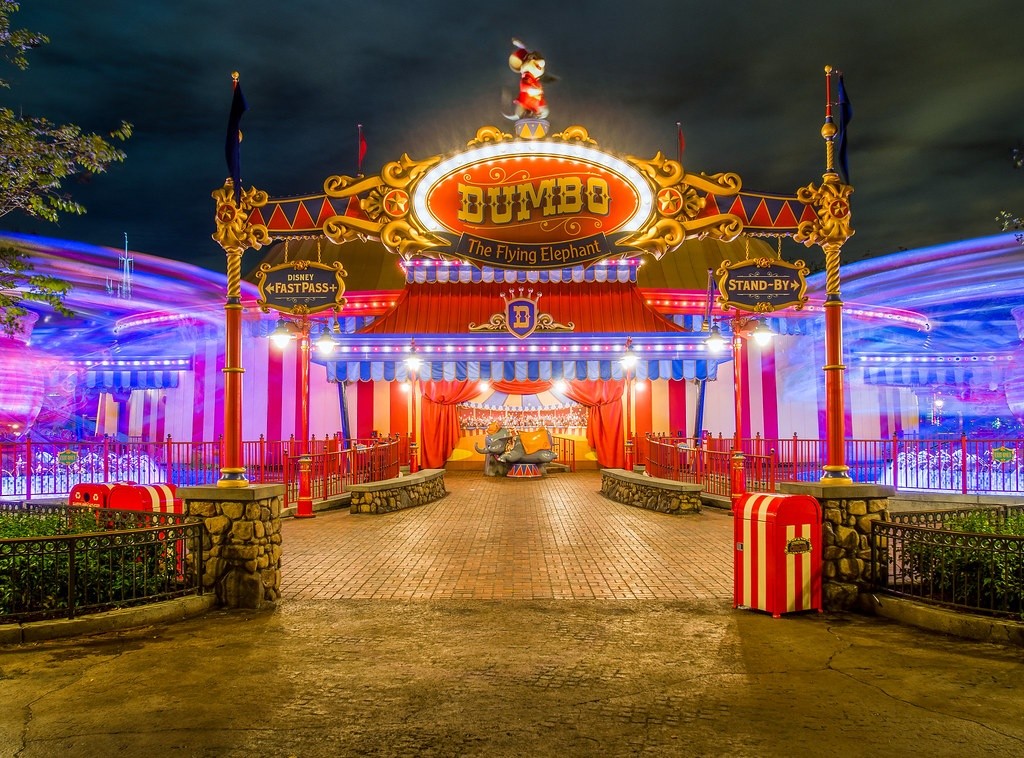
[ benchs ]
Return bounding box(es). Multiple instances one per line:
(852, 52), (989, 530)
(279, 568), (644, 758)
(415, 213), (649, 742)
(345, 469), (447, 515)
(599, 468), (705, 517)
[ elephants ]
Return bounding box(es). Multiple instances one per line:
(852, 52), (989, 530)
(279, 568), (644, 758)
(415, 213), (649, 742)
(474, 421), (557, 476)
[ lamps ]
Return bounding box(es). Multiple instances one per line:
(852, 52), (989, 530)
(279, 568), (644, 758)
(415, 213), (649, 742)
(703, 308), (779, 353)
(266, 311), (340, 355)
